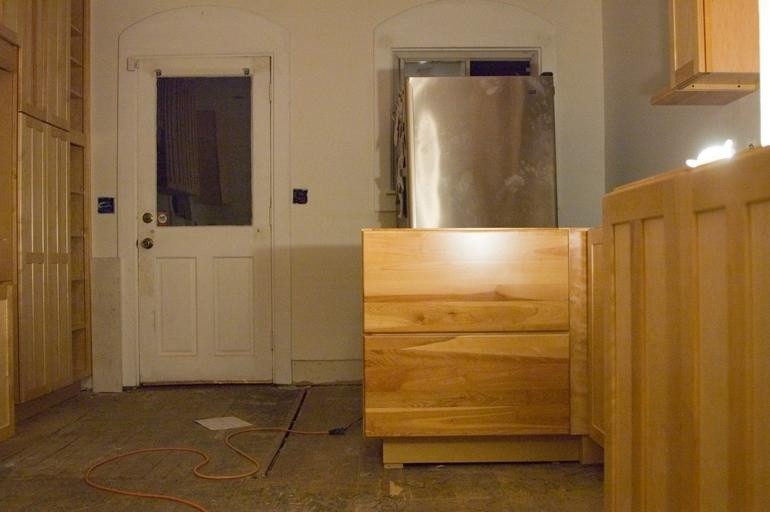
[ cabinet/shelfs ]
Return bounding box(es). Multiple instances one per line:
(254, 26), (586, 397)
(649, 1), (760, 107)
